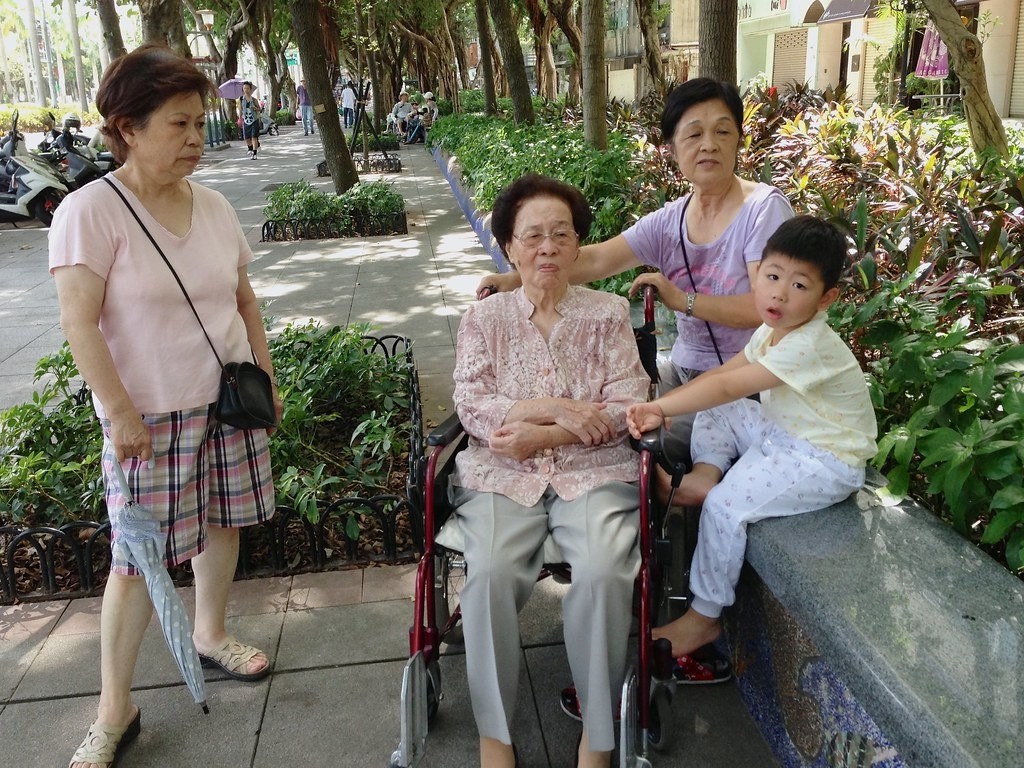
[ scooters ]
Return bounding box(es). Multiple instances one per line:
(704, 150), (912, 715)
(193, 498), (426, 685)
(258, 105), (280, 137)
(0, 109), (117, 230)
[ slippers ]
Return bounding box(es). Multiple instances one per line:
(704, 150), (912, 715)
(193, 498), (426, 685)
(670, 651), (732, 684)
(560, 685), (639, 723)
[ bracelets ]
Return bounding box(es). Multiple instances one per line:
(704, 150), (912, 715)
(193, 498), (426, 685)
(270, 377), (278, 386)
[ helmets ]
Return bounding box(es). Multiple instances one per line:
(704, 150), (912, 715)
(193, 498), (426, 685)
(62, 112), (81, 130)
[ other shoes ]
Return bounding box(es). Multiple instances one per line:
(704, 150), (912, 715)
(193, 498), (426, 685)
(401, 131), (425, 144)
(304, 133), (308, 136)
(251, 152), (257, 159)
(257, 142), (260, 148)
(311, 130), (314, 134)
(247, 150), (253, 155)
(345, 125), (347, 128)
(349, 125), (352, 128)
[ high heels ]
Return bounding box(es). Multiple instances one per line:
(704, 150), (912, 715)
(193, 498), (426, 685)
(195, 635), (270, 681)
(68, 703), (141, 768)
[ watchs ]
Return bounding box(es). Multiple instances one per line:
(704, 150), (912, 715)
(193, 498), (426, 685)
(685, 292), (697, 317)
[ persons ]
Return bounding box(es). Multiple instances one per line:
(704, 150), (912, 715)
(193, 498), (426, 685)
(237, 81), (261, 159)
(627, 214), (878, 657)
(454, 171), (651, 768)
(295, 77), (315, 136)
(47, 43), (281, 768)
(477, 75), (798, 725)
(391, 91), (438, 145)
(338, 81), (357, 129)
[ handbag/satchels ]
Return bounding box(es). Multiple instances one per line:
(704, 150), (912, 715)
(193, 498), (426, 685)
(215, 361), (276, 430)
(237, 117), (244, 128)
(296, 107), (302, 119)
(339, 106), (343, 116)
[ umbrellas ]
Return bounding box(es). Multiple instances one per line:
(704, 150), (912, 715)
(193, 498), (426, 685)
(219, 78), (257, 100)
(113, 448), (211, 715)
(914, 19), (950, 113)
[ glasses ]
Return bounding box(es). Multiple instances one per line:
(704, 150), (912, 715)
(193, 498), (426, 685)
(510, 229), (580, 248)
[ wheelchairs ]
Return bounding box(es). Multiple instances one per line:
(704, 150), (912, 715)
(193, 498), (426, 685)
(389, 280), (689, 767)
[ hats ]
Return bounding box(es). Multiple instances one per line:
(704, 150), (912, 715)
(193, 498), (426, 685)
(300, 77), (305, 82)
(423, 92), (433, 99)
(399, 91), (409, 100)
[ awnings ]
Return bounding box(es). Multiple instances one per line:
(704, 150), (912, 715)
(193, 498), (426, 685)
(816, 0), (873, 24)
